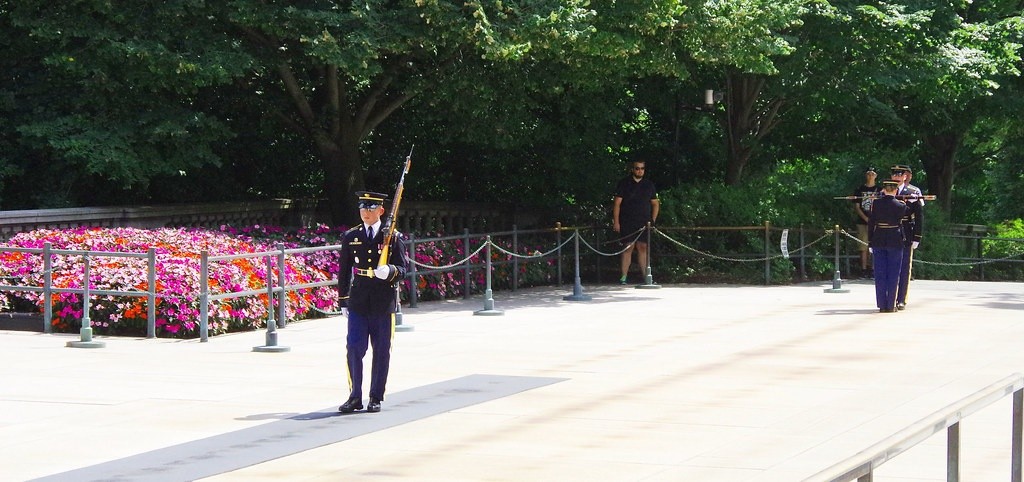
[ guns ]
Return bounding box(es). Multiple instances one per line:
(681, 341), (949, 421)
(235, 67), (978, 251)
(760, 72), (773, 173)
(376, 142), (415, 267)
(832, 194), (938, 201)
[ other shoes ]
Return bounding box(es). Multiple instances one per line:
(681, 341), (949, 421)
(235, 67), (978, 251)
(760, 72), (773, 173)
(897, 302), (904, 310)
(652, 279), (657, 285)
(880, 308), (893, 312)
(620, 275), (627, 285)
(367, 396), (380, 412)
(339, 396), (364, 413)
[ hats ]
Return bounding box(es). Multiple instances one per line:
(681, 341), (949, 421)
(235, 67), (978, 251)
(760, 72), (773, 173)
(890, 165), (912, 174)
(355, 191), (388, 209)
(883, 180), (898, 187)
(866, 166), (876, 174)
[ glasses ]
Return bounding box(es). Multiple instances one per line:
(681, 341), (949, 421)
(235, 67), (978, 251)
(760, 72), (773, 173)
(634, 166), (645, 170)
(360, 208), (376, 212)
(891, 173), (902, 177)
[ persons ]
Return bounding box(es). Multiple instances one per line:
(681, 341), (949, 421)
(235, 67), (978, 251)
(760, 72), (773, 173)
(868, 178), (910, 313)
(853, 163), (885, 280)
(613, 157), (659, 285)
(338, 191), (406, 412)
(881, 165), (924, 310)
(904, 165), (925, 206)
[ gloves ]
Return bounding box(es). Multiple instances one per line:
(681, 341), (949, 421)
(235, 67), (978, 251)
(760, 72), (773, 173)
(341, 308), (349, 321)
(911, 240), (919, 249)
(649, 218), (655, 226)
(868, 248), (875, 254)
(374, 265), (390, 280)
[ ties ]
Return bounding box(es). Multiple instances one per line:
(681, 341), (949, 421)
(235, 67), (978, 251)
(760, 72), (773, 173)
(368, 226), (373, 240)
